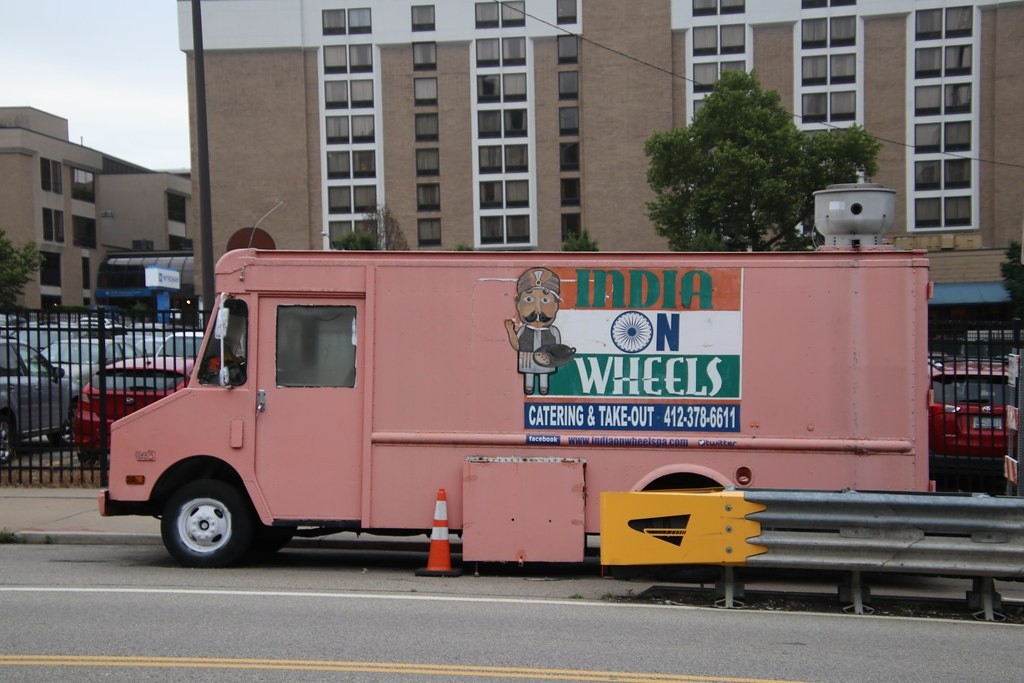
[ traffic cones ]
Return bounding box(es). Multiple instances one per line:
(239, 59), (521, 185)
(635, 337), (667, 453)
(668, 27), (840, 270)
(415, 488), (464, 576)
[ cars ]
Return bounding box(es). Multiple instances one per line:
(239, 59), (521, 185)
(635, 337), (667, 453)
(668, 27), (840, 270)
(0, 317), (205, 464)
(928, 327), (1024, 477)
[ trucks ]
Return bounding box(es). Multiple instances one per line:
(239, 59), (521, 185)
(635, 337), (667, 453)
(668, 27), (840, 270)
(98, 244), (937, 584)
